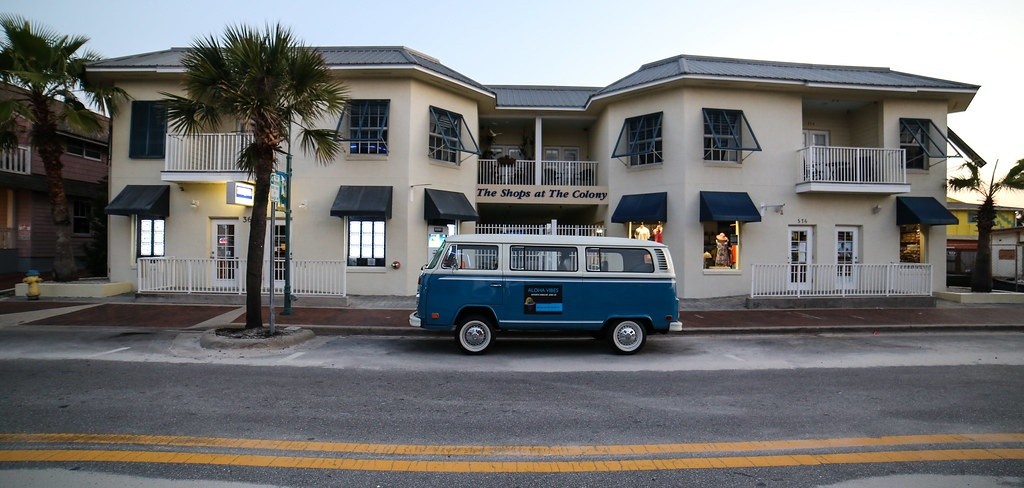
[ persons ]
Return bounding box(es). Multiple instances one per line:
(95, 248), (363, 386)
(634, 225), (663, 244)
(716, 233), (730, 266)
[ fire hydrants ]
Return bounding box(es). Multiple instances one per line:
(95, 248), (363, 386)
(23, 267), (44, 301)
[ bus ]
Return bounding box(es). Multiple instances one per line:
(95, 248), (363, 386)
(409, 234), (684, 356)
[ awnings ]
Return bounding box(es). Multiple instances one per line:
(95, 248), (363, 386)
(105, 184), (170, 220)
(611, 191), (668, 225)
(330, 185), (393, 220)
(699, 190), (762, 222)
(896, 196), (959, 227)
(424, 188), (479, 223)
